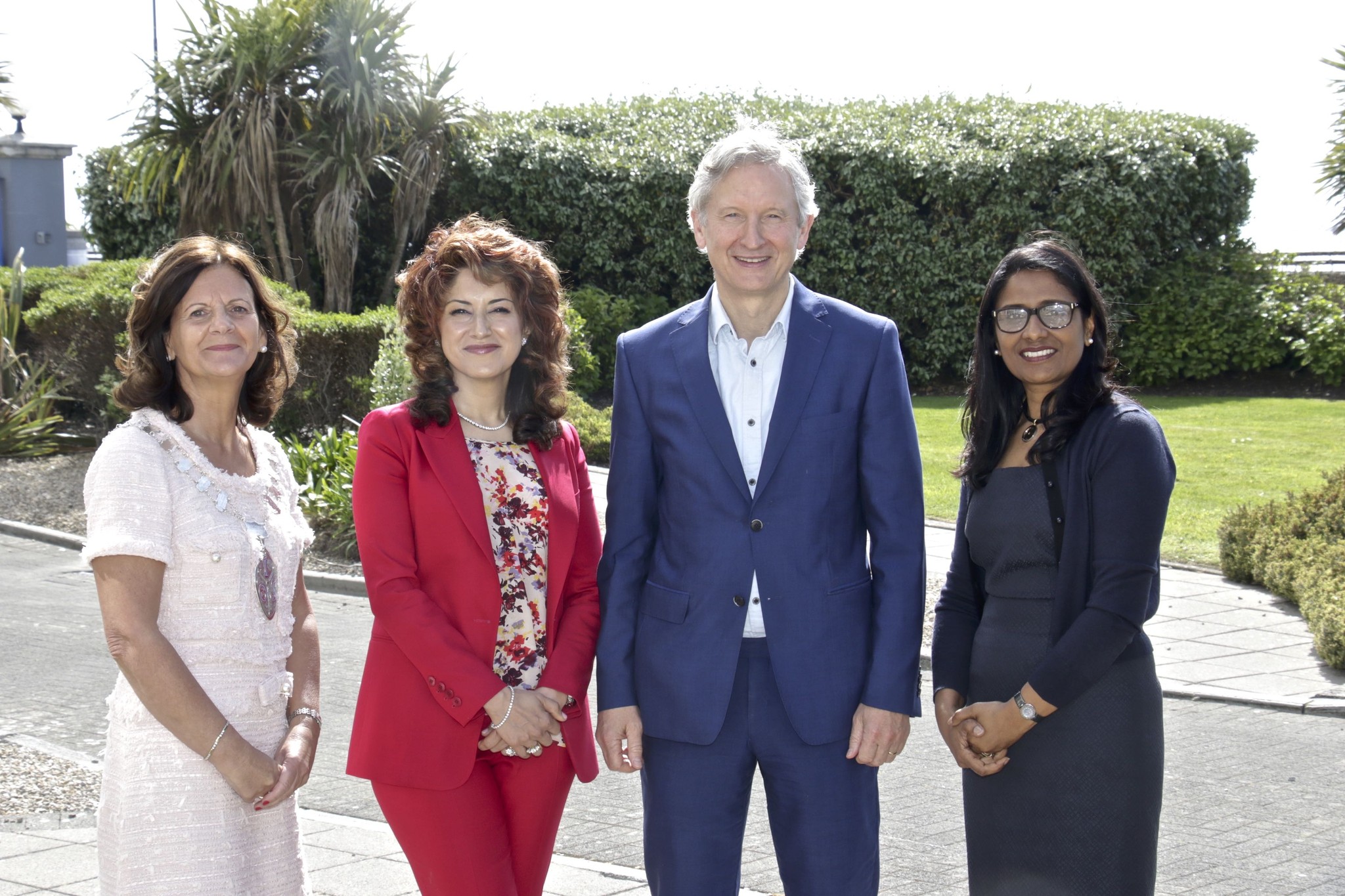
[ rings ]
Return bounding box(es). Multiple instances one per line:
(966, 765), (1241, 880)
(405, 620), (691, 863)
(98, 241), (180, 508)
(526, 742), (543, 755)
(888, 751), (898, 755)
(504, 745), (518, 758)
(982, 751), (995, 758)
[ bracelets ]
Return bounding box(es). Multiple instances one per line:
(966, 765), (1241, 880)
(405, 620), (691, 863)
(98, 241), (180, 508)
(203, 718), (231, 762)
(490, 685), (514, 729)
(566, 694), (574, 707)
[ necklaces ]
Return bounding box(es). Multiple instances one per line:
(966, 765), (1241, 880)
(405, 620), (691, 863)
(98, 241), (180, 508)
(1021, 399), (1059, 442)
(101, 407), (282, 619)
(456, 410), (512, 432)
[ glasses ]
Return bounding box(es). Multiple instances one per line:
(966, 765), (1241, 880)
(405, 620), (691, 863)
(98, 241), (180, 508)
(991, 303), (1079, 333)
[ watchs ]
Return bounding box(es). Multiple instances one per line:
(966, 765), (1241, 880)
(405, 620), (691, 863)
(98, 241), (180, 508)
(82, 235), (322, 896)
(1014, 691), (1050, 725)
(286, 708), (323, 729)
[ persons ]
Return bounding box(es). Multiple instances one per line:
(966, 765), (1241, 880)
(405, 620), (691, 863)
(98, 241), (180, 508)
(345, 217), (605, 896)
(594, 126), (926, 896)
(931, 241), (1176, 896)
